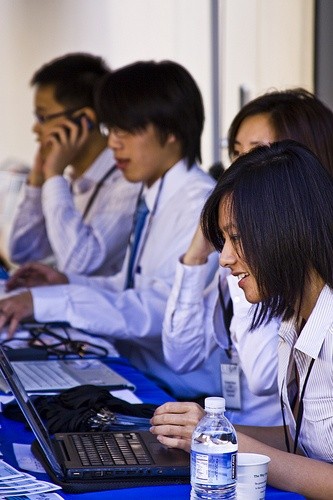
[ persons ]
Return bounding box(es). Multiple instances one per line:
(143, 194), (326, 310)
(0, 61), (230, 408)
(147, 141), (333, 500)
(160, 88), (333, 426)
(8, 52), (143, 278)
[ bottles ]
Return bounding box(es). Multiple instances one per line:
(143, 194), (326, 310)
(191, 397), (238, 500)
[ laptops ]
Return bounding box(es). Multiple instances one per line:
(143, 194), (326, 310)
(0, 348), (190, 480)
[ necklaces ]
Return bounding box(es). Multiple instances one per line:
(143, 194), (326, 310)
(280, 319), (317, 454)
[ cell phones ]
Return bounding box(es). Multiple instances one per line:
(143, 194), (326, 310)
(51, 113), (96, 142)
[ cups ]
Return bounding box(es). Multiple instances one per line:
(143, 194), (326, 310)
(237, 453), (271, 500)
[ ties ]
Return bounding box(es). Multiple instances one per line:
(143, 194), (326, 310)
(122, 197), (149, 291)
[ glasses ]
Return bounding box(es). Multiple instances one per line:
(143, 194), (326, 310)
(29, 322), (108, 361)
(100, 124), (128, 138)
(34, 108), (75, 123)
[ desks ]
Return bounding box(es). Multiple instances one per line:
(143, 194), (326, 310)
(0, 268), (310, 500)
